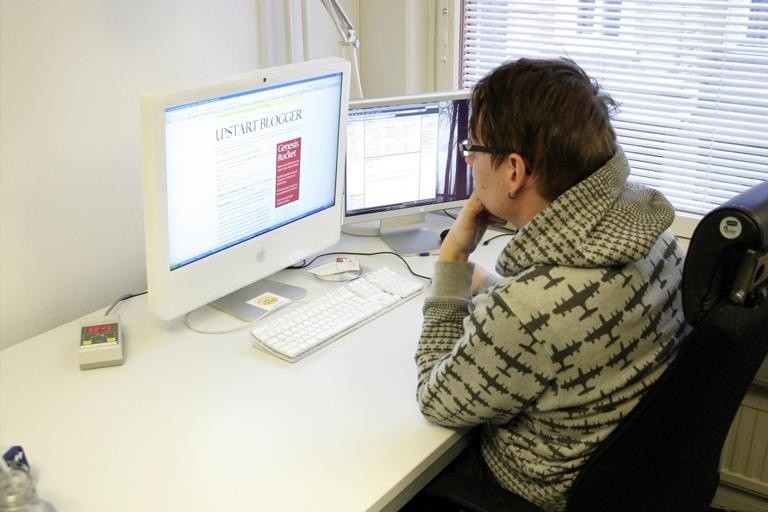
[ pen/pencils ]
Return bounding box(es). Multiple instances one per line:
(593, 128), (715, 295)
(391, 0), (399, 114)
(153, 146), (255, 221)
(420, 252), (439, 256)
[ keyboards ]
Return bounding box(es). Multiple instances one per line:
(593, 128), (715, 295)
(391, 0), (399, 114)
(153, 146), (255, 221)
(247, 265), (424, 364)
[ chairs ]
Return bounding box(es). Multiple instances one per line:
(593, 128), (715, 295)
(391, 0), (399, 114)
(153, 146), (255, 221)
(564, 182), (768, 511)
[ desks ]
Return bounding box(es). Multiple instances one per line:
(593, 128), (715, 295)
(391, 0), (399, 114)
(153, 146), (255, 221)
(0, 214), (516, 512)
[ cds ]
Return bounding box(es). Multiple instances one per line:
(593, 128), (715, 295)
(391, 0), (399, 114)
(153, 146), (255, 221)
(316, 266), (363, 282)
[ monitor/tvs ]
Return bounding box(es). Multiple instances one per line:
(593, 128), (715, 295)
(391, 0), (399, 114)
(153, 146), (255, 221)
(339, 89), (475, 256)
(139, 57), (352, 325)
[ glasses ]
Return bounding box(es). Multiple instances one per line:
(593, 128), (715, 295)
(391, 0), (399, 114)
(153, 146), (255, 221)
(459, 138), (508, 158)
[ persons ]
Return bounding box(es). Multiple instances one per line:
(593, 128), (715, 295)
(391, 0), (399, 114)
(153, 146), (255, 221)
(416, 59), (692, 512)
